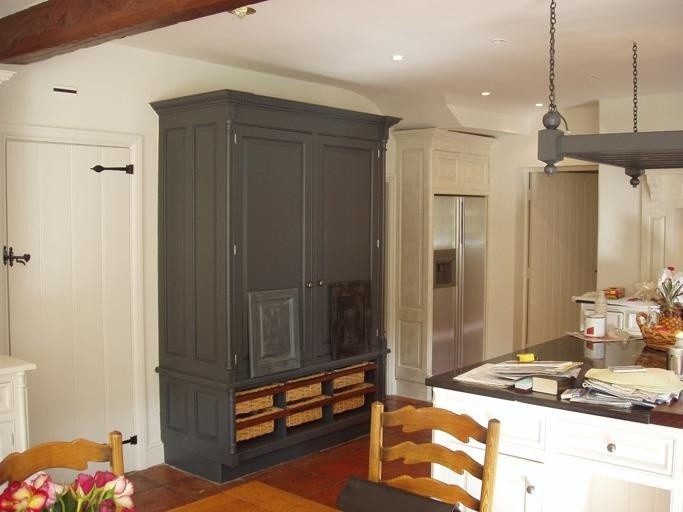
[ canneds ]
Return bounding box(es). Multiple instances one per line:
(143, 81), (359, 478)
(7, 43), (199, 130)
(584, 314), (606, 338)
(583, 340), (605, 361)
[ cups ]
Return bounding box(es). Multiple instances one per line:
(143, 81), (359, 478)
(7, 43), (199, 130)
(666, 345), (683, 376)
(581, 314), (606, 339)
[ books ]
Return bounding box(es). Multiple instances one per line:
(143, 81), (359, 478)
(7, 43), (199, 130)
(451, 359), (682, 410)
(566, 324), (644, 344)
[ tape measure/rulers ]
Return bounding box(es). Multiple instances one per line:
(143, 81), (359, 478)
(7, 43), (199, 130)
(515, 352), (540, 362)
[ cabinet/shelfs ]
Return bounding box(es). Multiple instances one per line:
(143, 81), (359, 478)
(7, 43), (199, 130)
(0, 355), (37, 460)
(150, 90), (404, 485)
(432, 387), (683, 512)
(570, 296), (661, 340)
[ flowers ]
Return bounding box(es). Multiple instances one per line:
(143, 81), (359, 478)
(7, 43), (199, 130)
(0, 471), (135, 512)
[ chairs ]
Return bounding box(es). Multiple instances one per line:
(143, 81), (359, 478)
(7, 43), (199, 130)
(368, 400), (501, 512)
(0, 431), (124, 485)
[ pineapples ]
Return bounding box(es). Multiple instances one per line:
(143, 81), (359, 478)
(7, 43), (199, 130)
(652, 278), (683, 333)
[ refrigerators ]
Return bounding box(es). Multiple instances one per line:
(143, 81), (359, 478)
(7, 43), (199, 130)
(432, 193), (484, 375)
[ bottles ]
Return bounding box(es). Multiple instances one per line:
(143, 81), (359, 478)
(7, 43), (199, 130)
(595, 291), (607, 316)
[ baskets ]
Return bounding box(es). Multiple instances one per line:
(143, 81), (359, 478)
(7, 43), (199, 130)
(636, 311), (682, 352)
